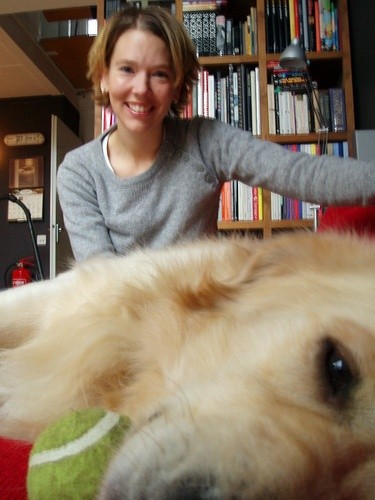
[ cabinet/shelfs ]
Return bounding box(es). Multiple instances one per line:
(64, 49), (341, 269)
(95, 0), (363, 243)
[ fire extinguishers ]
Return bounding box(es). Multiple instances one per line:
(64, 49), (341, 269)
(3, 256), (36, 289)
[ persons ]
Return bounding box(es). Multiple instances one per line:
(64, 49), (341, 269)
(57, 5), (375, 265)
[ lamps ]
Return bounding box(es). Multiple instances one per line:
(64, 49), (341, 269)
(278, 37), (330, 233)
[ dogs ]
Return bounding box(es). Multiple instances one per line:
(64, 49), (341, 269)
(0, 223), (374, 500)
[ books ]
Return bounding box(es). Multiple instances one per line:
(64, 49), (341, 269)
(100, 0), (351, 222)
